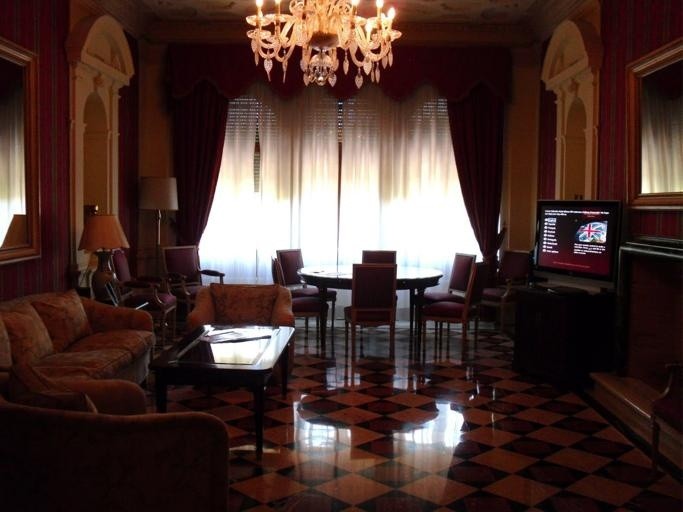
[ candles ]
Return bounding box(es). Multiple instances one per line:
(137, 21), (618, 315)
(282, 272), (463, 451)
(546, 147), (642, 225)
(275, 1), (280, 17)
(389, 20), (393, 30)
(258, 7), (261, 19)
(352, 4), (357, 16)
(377, 7), (381, 19)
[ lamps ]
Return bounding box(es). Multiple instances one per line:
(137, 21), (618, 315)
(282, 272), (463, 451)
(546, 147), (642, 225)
(245, 0), (402, 88)
(135, 175), (178, 210)
(77, 214), (130, 302)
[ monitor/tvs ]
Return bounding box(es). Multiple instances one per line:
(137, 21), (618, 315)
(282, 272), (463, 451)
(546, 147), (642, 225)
(529, 198), (624, 294)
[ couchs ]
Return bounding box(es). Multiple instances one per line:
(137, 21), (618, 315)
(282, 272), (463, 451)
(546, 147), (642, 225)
(0, 319), (230, 512)
(0, 289), (155, 385)
(186, 283), (295, 335)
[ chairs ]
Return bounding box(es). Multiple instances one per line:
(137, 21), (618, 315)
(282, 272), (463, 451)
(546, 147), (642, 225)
(481, 249), (532, 334)
(160, 246), (225, 314)
(276, 249), (337, 331)
(422, 262), (486, 354)
(271, 256), (329, 351)
(361, 250), (397, 265)
(111, 248), (177, 347)
(344, 264), (397, 360)
(414, 253), (477, 329)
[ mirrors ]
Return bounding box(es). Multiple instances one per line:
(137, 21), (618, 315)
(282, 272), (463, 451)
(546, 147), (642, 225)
(0, 38), (41, 264)
(624, 36), (683, 214)
